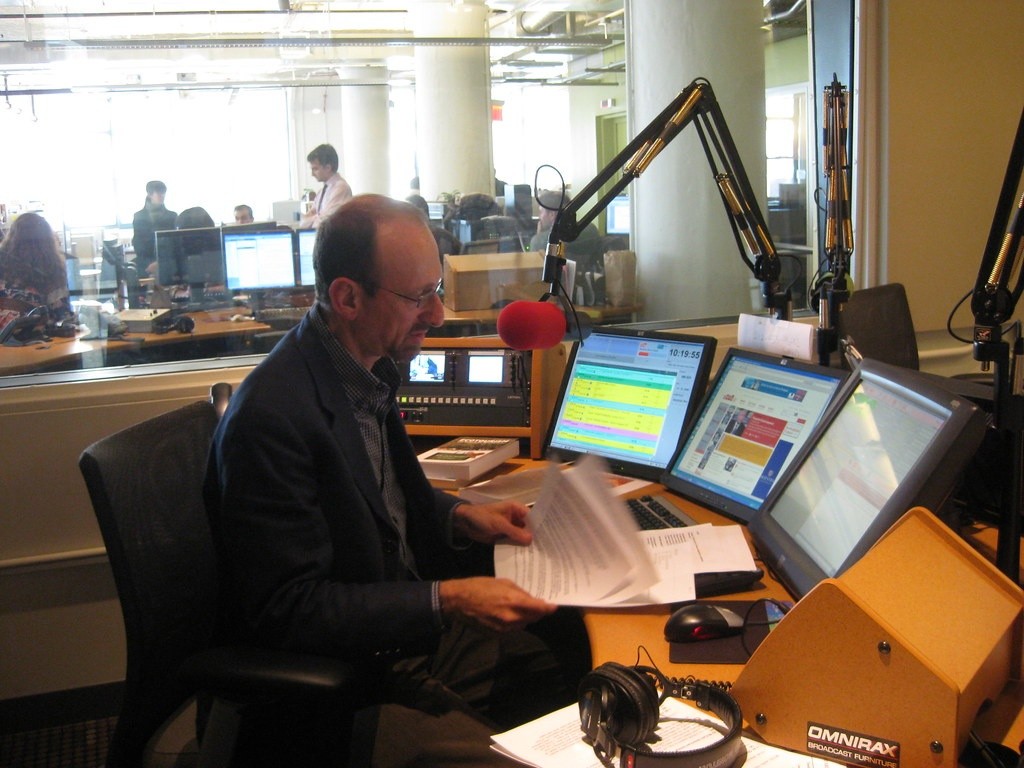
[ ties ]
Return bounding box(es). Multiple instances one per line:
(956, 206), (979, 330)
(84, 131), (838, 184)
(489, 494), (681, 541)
(318, 184), (328, 211)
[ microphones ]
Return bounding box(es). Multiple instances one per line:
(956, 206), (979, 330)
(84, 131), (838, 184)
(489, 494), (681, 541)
(543, 225), (558, 283)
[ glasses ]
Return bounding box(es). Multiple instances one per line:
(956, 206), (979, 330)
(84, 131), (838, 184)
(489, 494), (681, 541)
(234, 216), (248, 221)
(342, 271), (444, 309)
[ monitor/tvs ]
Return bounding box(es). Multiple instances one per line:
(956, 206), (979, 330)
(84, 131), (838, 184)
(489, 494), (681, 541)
(155, 202), (532, 313)
(409, 354), (445, 382)
(468, 355), (503, 383)
(542, 325), (986, 597)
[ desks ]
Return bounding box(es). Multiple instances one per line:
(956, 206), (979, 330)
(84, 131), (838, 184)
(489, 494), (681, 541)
(0, 305), (272, 377)
(440, 456), (1023, 768)
(441, 307), (633, 324)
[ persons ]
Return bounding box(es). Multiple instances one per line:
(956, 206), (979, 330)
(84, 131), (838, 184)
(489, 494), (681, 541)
(202, 195), (590, 767)
(0, 213), (78, 329)
(406, 195), (430, 217)
(300, 143), (352, 229)
(130, 180), (180, 278)
(408, 177), (420, 195)
(174, 207), (215, 230)
(527, 190), (603, 272)
(233, 205), (254, 225)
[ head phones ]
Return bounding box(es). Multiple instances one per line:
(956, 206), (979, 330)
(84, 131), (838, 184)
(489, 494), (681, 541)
(576, 661), (742, 768)
(151, 315), (195, 334)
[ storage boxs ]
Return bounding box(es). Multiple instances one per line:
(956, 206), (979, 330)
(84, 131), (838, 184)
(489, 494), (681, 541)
(116, 309), (172, 333)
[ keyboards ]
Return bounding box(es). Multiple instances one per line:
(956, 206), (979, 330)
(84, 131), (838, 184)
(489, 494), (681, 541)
(625, 493), (764, 596)
(255, 306), (313, 323)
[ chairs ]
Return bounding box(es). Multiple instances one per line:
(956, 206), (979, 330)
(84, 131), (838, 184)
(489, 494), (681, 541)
(434, 227), (500, 256)
(78, 398), (361, 768)
(838, 284), (920, 376)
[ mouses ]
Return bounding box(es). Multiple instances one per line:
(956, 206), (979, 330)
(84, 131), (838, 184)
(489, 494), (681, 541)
(663, 603), (746, 644)
